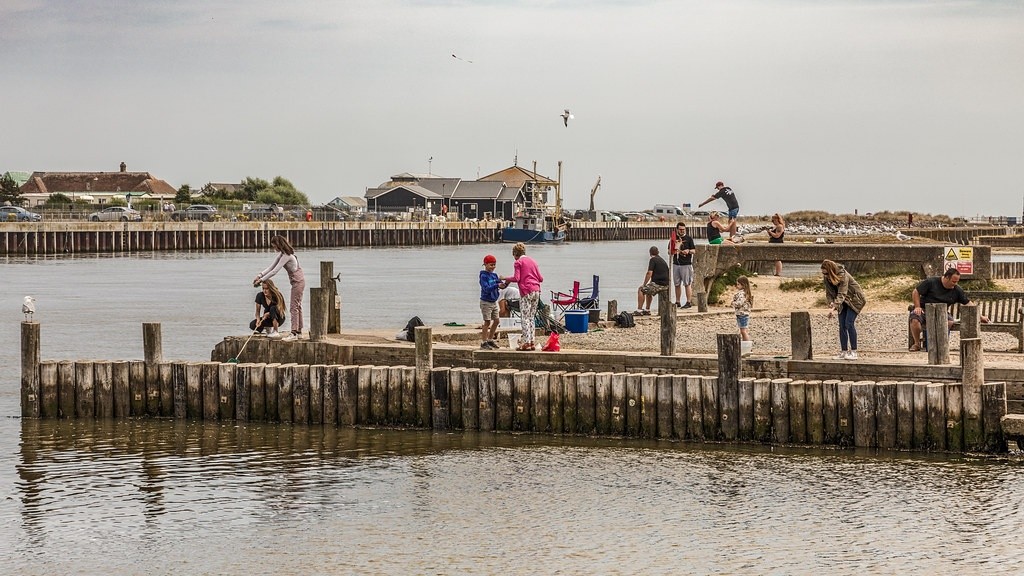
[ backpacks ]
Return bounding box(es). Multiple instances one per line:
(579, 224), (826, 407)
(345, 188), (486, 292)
(619, 311), (634, 328)
(403, 316), (425, 341)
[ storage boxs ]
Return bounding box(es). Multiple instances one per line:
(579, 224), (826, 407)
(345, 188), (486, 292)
(564, 309), (589, 333)
(499, 318), (523, 331)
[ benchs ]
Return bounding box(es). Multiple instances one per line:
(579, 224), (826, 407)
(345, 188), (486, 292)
(907, 290), (1024, 353)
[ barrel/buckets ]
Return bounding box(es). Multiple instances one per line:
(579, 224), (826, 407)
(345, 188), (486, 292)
(508, 334), (521, 348)
(741, 341), (752, 356)
(588, 309), (600, 322)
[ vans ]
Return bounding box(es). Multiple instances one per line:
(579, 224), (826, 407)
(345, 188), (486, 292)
(652, 204), (687, 222)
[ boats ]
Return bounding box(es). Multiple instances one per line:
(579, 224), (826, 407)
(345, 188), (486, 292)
(500, 160), (569, 245)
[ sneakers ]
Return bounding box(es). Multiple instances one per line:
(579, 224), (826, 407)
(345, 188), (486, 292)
(480, 342), (492, 350)
(488, 340), (499, 349)
(845, 351), (858, 360)
(832, 350), (847, 360)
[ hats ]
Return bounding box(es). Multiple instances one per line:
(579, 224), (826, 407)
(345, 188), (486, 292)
(484, 255), (496, 264)
(715, 182), (723, 189)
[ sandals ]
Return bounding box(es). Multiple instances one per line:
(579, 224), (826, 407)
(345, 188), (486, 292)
(516, 342), (535, 351)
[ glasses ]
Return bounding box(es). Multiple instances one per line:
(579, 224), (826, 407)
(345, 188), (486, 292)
(772, 218), (775, 222)
(822, 272), (828, 275)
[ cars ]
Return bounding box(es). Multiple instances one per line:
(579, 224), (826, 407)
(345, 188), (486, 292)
(0, 206), (41, 222)
(88, 207), (141, 222)
(572, 209), (661, 222)
(695, 211), (727, 222)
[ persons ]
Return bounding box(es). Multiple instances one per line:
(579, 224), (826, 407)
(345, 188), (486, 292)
(821, 260), (867, 360)
(305, 208), (312, 222)
(707, 211), (734, 244)
(730, 275), (753, 341)
(498, 242), (543, 351)
(699, 182), (740, 240)
(164, 202), (169, 211)
(250, 279), (286, 338)
(497, 286), (521, 318)
(908, 268), (989, 351)
(168, 202), (176, 212)
(479, 255), (510, 350)
(634, 246), (669, 316)
(766, 213), (785, 276)
(253, 236), (305, 342)
(668, 222), (696, 309)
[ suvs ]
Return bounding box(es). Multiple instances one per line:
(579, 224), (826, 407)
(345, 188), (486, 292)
(171, 204), (219, 221)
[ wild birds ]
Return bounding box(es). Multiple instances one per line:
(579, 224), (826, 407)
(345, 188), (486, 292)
(22, 295), (36, 323)
(732, 222), (914, 243)
(559, 107), (575, 127)
(452, 54), (473, 64)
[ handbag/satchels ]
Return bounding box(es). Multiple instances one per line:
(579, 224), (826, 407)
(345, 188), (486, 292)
(542, 332), (560, 351)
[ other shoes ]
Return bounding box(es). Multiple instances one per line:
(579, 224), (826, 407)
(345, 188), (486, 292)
(266, 327), (274, 336)
(675, 302), (681, 309)
(681, 303), (692, 310)
(910, 342), (920, 351)
(632, 310), (643, 316)
(281, 333), (298, 341)
(642, 311), (651, 315)
(267, 330), (280, 337)
(297, 334), (303, 339)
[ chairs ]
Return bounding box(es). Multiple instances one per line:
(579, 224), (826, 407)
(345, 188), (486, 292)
(549, 275), (601, 322)
(509, 298), (523, 319)
